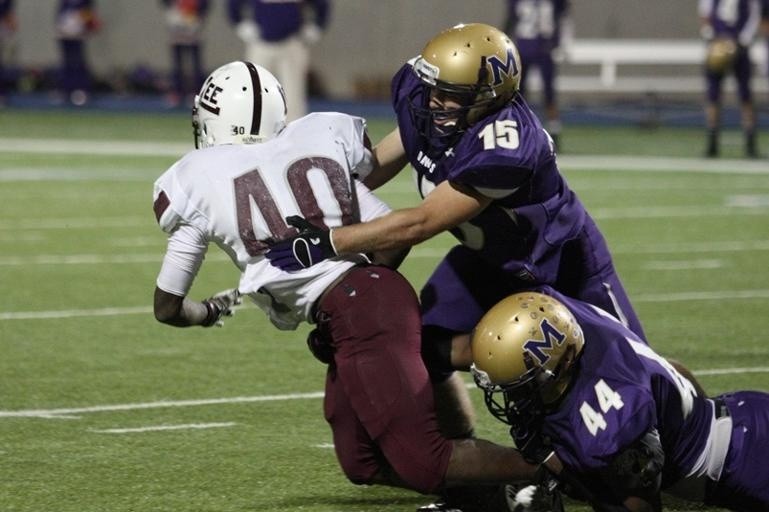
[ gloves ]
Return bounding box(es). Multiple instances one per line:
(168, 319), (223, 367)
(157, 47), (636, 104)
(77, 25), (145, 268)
(264, 215), (339, 272)
(198, 288), (243, 327)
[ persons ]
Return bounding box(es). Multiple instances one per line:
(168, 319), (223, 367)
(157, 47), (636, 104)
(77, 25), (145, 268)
(52, 1), (102, 105)
(262, 23), (653, 510)
(228, 0), (331, 131)
(695, 0), (764, 161)
(502, 0), (573, 143)
(158, 2), (214, 112)
(307, 288), (767, 511)
(2, 1), (20, 87)
(148, 55), (543, 512)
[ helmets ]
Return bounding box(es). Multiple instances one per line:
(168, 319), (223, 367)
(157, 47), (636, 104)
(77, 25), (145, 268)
(192, 61), (288, 148)
(469, 293), (586, 426)
(405, 22), (522, 139)
